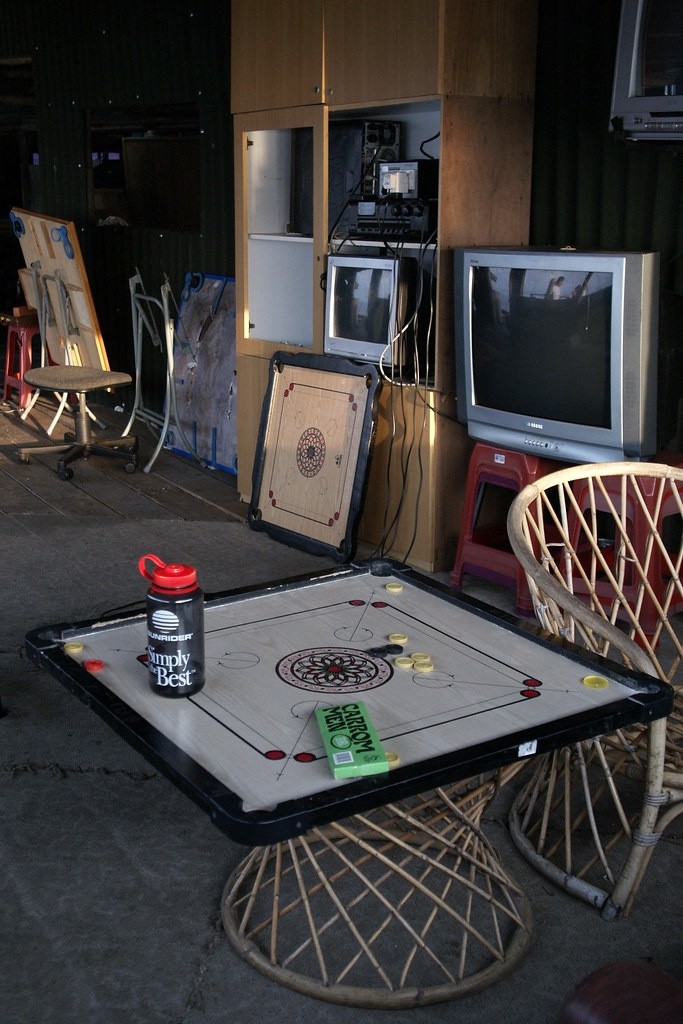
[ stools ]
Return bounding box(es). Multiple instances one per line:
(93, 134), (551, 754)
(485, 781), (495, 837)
(1, 323), (79, 409)
(451, 441), (575, 617)
(559, 451), (683, 656)
(18, 365), (142, 481)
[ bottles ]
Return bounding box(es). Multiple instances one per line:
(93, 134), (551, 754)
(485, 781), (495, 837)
(138, 553), (207, 699)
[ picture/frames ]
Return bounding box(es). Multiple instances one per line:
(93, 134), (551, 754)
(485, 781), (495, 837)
(248, 350), (383, 565)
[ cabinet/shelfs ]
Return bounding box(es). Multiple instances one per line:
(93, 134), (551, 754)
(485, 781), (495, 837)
(230, 0), (532, 574)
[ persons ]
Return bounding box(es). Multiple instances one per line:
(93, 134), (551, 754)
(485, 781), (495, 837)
(550, 276), (565, 299)
(572, 284), (585, 297)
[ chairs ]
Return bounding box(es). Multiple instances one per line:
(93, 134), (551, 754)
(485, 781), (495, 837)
(502, 461), (683, 919)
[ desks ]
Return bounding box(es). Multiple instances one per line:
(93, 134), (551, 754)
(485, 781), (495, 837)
(11, 206), (115, 434)
(27, 558), (675, 1011)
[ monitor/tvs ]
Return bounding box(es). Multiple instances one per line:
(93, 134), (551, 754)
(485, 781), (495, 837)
(322, 252), (435, 377)
(608, 0), (683, 142)
(452, 242), (665, 472)
